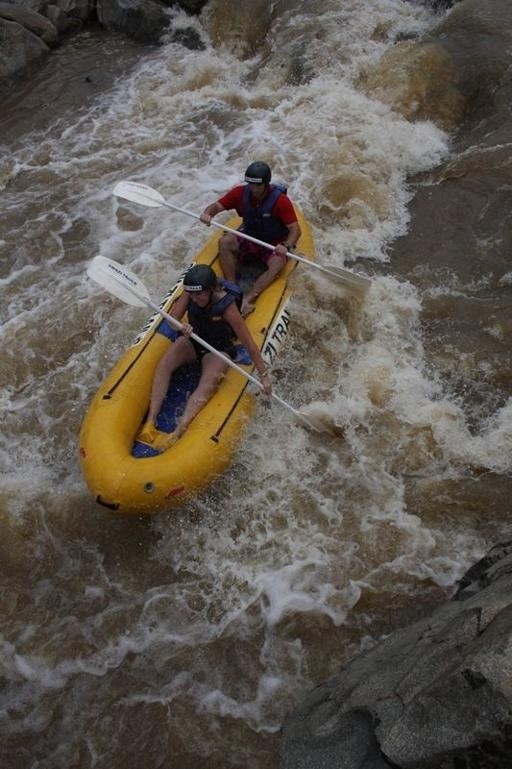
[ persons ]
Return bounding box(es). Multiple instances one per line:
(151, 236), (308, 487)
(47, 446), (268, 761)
(198, 160), (301, 312)
(138, 263), (275, 455)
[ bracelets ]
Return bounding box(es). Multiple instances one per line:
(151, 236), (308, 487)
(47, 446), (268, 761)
(258, 370), (269, 379)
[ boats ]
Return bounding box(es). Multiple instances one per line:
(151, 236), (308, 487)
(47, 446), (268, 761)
(75, 197), (315, 516)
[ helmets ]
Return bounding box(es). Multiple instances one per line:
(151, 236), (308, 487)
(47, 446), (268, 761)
(244, 161), (271, 184)
(183, 264), (216, 293)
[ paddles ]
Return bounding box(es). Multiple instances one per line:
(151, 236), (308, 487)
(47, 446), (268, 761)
(86, 255), (337, 438)
(113, 180), (372, 298)
